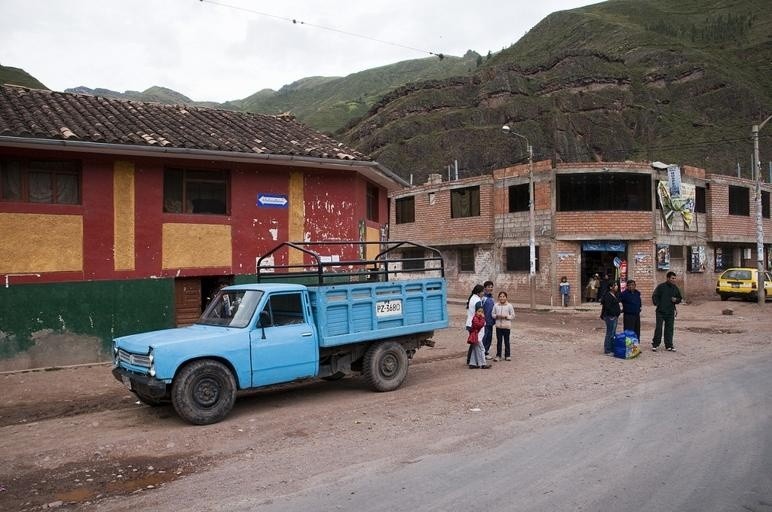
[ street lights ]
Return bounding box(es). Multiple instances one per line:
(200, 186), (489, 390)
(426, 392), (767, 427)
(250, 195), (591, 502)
(500, 124), (537, 310)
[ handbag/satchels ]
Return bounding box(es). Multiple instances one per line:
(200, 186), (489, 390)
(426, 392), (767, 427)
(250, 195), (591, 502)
(611, 329), (643, 360)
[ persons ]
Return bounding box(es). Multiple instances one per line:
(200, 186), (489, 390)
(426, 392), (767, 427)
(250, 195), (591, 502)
(491, 291), (516, 362)
(583, 272), (600, 302)
(619, 279), (643, 344)
(598, 274), (611, 301)
(650, 271), (683, 352)
(468, 306), (493, 369)
(559, 276), (570, 307)
(465, 284), (486, 364)
(482, 281), (495, 360)
(600, 281), (621, 355)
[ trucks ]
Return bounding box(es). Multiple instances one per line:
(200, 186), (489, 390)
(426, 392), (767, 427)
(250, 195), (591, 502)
(104, 237), (448, 431)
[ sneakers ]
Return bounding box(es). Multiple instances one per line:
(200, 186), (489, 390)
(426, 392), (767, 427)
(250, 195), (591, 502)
(493, 355), (503, 363)
(664, 347), (676, 352)
(651, 346), (657, 352)
(463, 354), (493, 370)
(504, 356), (512, 361)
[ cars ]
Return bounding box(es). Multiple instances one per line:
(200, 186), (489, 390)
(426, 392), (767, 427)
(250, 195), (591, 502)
(714, 266), (772, 301)
